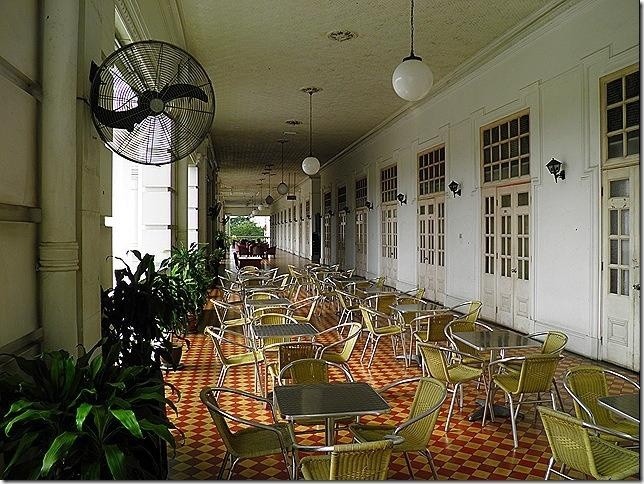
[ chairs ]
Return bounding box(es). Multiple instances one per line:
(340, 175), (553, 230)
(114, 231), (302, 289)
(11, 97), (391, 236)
(563, 366), (640, 452)
(536, 405), (640, 481)
(233, 238), (277, 268)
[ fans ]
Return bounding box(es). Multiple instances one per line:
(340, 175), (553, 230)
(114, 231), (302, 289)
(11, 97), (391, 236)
(210, 202), (222, 221)
(88, 39), (216, 166)
(218, 216), (230, 226)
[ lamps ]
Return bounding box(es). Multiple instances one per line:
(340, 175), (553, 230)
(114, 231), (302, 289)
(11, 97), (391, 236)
(248, 138), (290, 218)
(545, 158), (565, 184)
(392, 0), (434, 101)
(271, 214), (310, 225)
(300, 84), (323, 176)
(315, 179), (461, 219)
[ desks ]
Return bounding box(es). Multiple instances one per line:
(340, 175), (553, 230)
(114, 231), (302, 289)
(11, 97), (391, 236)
(596, 393), (640, 426)
